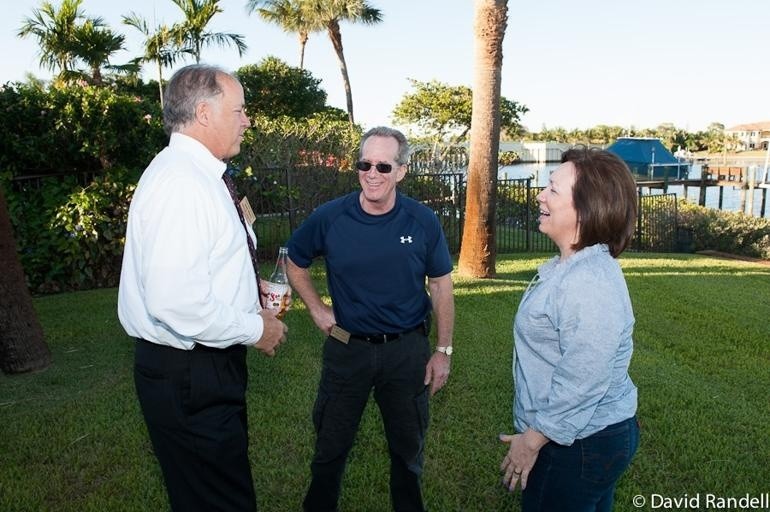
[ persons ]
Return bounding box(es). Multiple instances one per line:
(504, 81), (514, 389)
(285, 126), (456, 511)
(497, 145), (642, 511)
(115, 63), (292, 512)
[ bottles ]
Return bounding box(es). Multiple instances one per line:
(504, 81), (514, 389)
(265, 247), (292, 325)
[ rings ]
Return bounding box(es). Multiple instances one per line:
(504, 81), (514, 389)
(514, 470), (521, 475)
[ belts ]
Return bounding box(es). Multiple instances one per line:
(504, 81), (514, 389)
(334, 322), (426, 346)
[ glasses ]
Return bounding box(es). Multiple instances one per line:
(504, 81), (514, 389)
(355, 160), (402, 174)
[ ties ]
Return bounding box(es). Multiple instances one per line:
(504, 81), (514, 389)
(222, 172), (265, 312)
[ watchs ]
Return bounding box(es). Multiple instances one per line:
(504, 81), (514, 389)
(435, 344), (455, 355)
